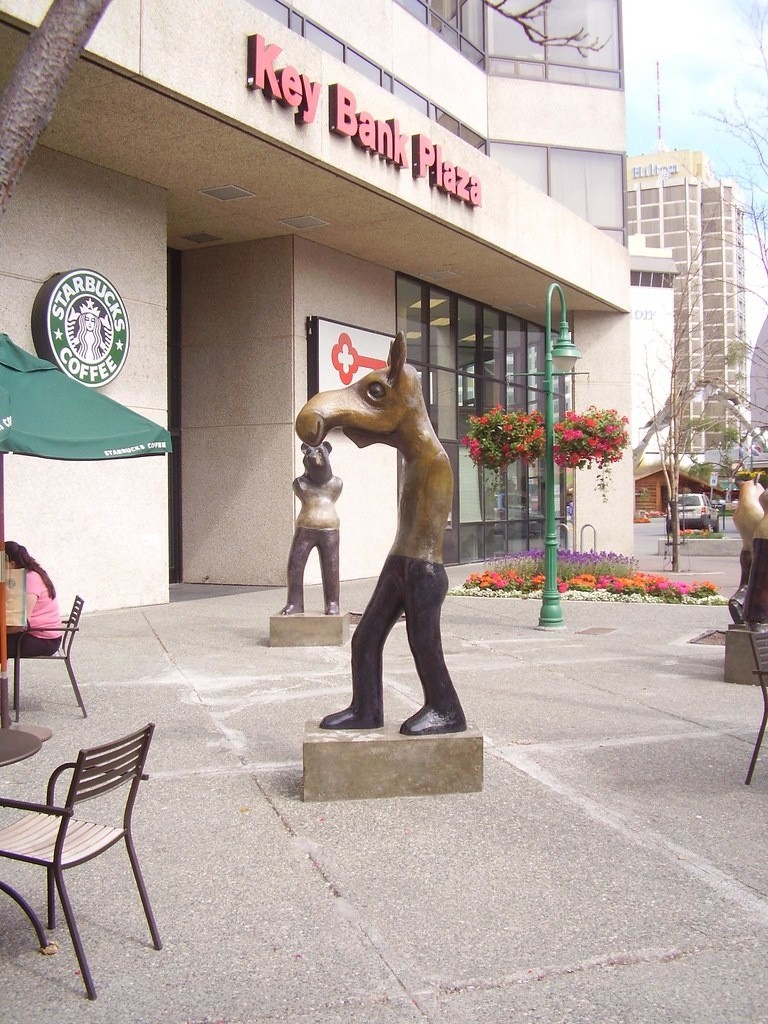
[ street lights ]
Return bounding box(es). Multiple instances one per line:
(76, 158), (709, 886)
(536, 282), (582, 627)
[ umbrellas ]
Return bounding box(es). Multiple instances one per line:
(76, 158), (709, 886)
(0, 334), (173, 730)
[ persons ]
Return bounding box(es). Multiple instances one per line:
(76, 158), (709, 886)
(0, 541), (63, 674)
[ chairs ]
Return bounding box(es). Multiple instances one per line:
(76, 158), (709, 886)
(14, 593), (87, 722)
(0, 722), (164, 1003)
(744, 632), (768, 784)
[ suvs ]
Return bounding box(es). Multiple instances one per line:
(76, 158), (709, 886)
(666, 493), (720, 534)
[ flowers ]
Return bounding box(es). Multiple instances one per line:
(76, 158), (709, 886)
(461, 403), (546, 499)
(552, 404), (631, 505)
